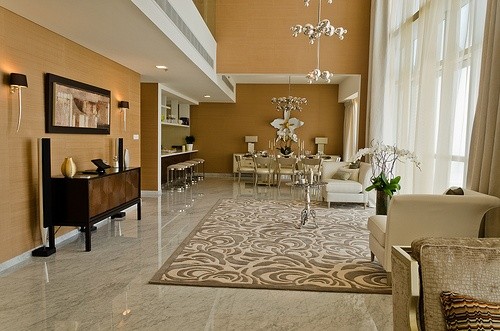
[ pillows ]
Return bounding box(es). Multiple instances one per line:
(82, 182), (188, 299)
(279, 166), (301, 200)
(332, 168), (352, 181)
(345, 168), (359, 182)
(443, 186), (465, 196)
(439, 290), (500, 331)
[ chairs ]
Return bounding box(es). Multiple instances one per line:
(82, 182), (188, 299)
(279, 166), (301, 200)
(235, 154), (340, 189)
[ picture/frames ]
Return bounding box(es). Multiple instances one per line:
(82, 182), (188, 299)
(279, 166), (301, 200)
(44, 73), (111, 135)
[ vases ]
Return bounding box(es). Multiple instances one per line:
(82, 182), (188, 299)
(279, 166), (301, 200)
(375, 189), (393, 215)
(60, 157), (78, 178)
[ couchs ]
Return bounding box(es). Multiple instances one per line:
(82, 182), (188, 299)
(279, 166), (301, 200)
(320, 159), (376, 208)
(366, 185), (500, 331)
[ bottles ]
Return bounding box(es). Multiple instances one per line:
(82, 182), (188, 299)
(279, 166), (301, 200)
(61, 157), (76, 177)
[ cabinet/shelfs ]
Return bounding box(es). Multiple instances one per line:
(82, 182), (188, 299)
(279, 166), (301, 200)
(161, 90), (199, 185)
(43, 137), (142, 254)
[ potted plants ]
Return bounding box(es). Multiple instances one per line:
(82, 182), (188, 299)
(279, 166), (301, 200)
(185, 135), (195, 151)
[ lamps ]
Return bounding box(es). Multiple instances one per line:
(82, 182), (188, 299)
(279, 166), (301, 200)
(271, 0), (348, 112)
(9, 72), (27, 133)
(119, 100), (130, 132)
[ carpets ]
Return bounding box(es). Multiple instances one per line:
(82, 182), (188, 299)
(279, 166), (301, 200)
(150, 197), (394, 295)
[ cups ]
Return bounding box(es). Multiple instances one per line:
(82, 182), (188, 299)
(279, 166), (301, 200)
(182, 145), (185, 152)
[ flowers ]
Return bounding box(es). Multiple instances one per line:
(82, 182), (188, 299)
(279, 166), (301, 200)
(270, 109), (304, 142)
(344, 136), (422, 198)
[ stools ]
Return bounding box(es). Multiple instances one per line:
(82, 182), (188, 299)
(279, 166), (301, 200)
(167, 159), (206, 193)
